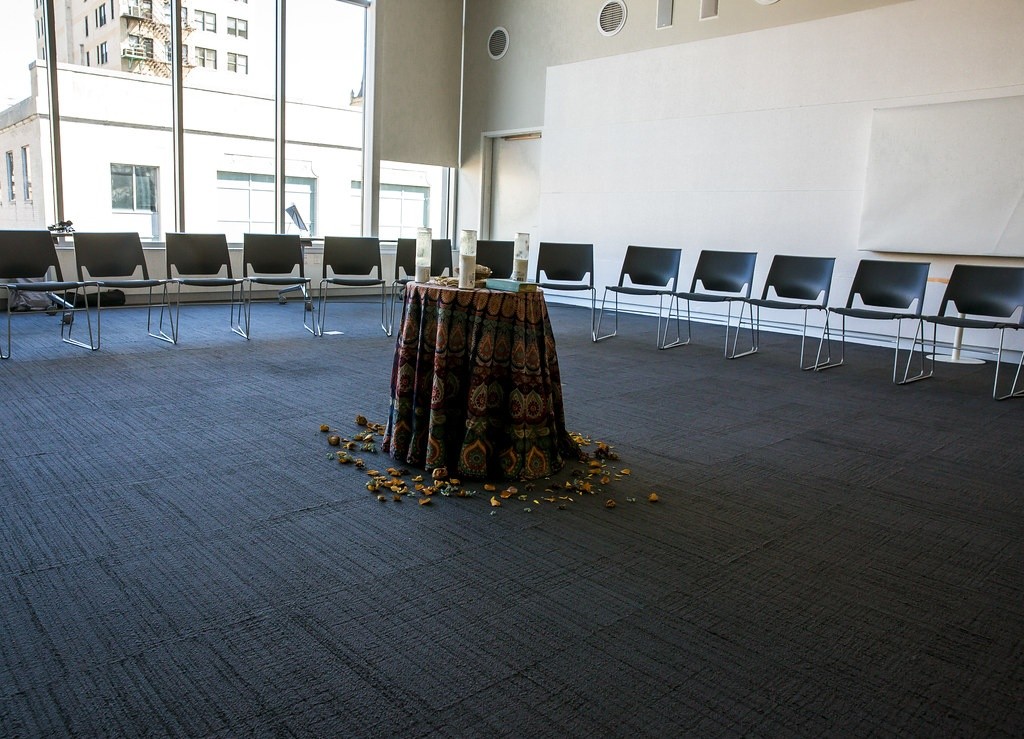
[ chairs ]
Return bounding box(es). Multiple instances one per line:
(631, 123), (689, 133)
(387, 239), (454, 336)
(476, 241), (514, 277)
(662, 251), (758, 358)
(316, 236), (390, 337)
(67, 232), (176, 351)
(813, 259), (930, 385)
(0, 229), (94, 360)
(595, 246), (682, 349)
(238, 233), (316, 336)
(535, 243), (596, 342)
(732, 256), (837, 370)
(160, 232), (248, 343)
(903, 264), (1024, 400)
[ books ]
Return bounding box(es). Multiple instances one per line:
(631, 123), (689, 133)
(487, 277), (539, 292)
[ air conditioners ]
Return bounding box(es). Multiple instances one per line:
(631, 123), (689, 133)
(129, 6), (140, 17)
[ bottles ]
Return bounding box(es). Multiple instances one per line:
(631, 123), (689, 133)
(512, 232), (530, 281)
(414, 227), (432, 283)
(458, 230), (477, 290)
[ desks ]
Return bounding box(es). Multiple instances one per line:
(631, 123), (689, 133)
(382, 281), (583, 484)
